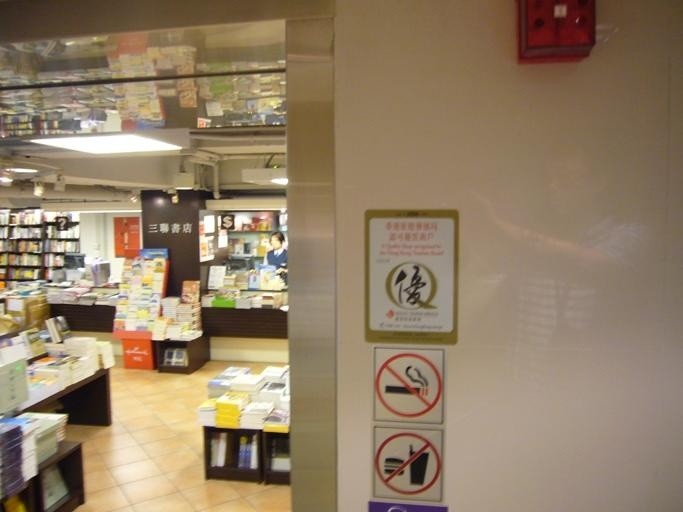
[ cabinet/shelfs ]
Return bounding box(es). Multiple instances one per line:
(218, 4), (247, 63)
(0, 215), (79, 283)
(226, 227), (272, 261)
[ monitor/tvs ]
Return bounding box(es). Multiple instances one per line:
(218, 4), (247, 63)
(64, 253), (85, 268)
(228, 253), (255, 271)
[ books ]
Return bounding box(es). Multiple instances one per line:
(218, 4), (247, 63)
(203, 263), (289, 310)
(193, 360), (290, 478)
(0, 345), (116, 511)
(199, 213), (269, 258)
(0, 208), (202, 345)
(156, 346), (188, 369)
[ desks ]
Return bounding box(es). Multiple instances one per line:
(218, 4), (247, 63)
(155, 335), (211, 374)
(18, 363), (112, 510)
(203, 425), (289, 488)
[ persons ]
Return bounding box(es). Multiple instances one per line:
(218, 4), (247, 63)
(260, 232), (289, 282)
